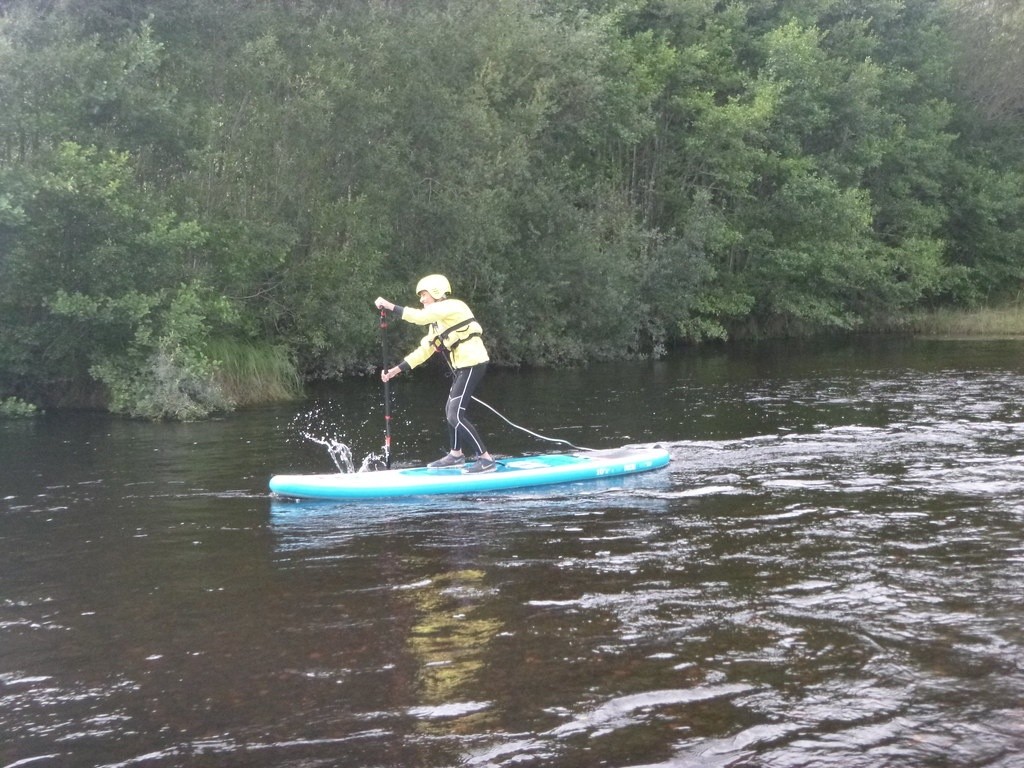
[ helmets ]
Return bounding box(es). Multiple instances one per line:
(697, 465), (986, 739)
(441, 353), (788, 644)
(415, 274), (452, 299)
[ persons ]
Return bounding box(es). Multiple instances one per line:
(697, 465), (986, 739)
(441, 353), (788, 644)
(375, 273), (496, 476)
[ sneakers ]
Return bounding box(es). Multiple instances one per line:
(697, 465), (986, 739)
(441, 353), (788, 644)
(427, 453), (466, 468)
(461, 454), (497, 474)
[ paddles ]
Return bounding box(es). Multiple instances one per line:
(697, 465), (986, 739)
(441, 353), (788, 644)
(267, 445), (671, 501)
(377, 304), (395, 469)
